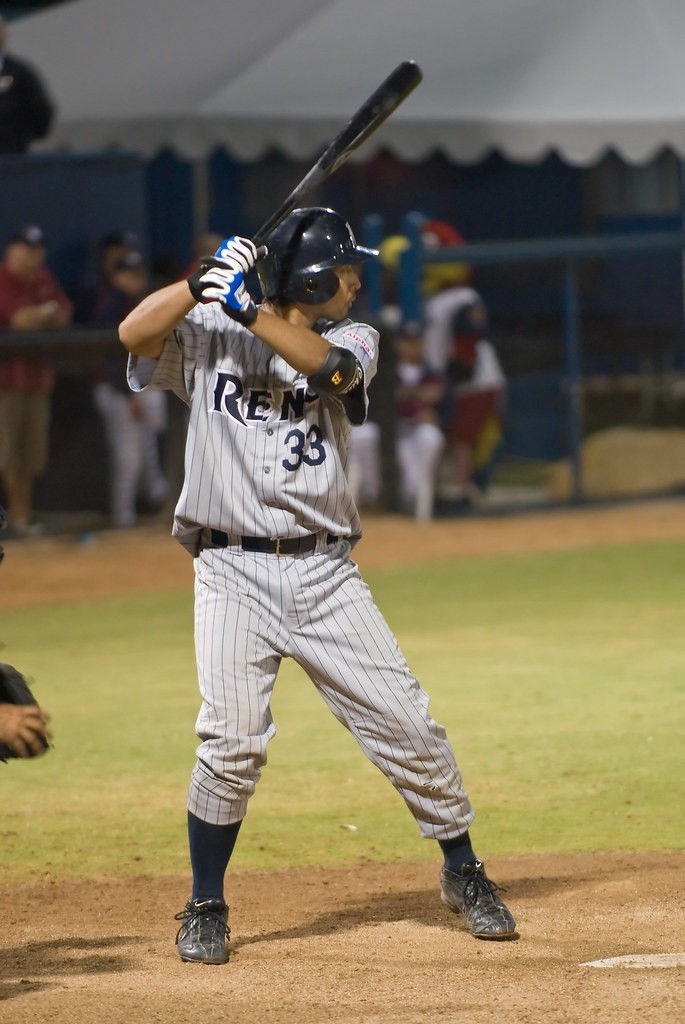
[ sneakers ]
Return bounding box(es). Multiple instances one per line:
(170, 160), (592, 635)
(439, 859), (516, 941)
(174, 898), (231, 964)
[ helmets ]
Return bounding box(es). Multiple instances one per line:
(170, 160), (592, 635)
(254, 207), (380, 304)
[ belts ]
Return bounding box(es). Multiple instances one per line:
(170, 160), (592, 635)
(211, 529), (347, 556)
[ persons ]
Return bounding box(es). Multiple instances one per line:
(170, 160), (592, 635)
(90, 223), (506, 528)
(0, 225), (73, 536)
(119, 206), (518, 964)
(0, 509), (52, 763)
(0, 15), (52, 155)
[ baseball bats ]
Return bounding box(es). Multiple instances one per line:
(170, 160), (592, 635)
(250, 59), (424, 249)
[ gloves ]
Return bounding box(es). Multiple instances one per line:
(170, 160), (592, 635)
(186, 236), (257, 304)
(199, 255), (258, 327)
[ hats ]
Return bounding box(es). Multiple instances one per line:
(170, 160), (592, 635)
(7, 225), (43, 246)
